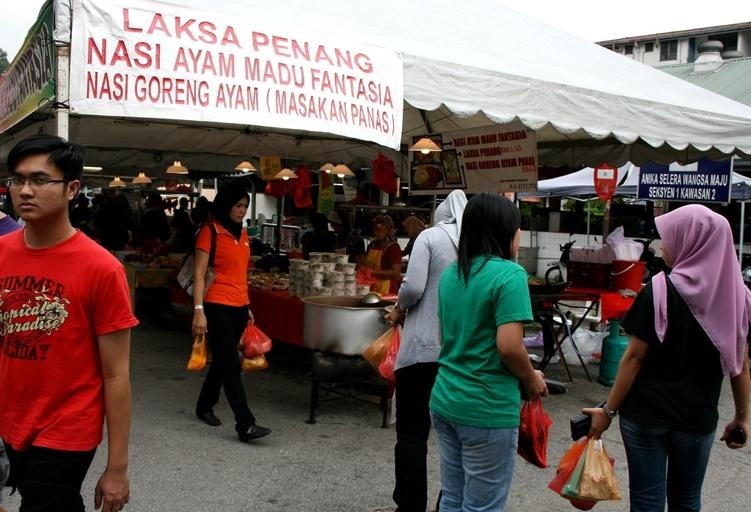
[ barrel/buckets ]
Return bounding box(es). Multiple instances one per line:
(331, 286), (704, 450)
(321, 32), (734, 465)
(610, 260), (647, 293)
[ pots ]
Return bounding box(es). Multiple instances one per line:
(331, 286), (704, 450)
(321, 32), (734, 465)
(300, 295), (397, 358)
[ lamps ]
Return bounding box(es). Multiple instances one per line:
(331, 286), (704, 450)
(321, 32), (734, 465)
(273, 168), (299, 182)
(407, 138), (443, 155)
(108, 175), (126, 188)
(165, 160), (190, 175)
(234, 161), (256, 173)
(130, 171), (153, 184)
(319, 162), (356, 179)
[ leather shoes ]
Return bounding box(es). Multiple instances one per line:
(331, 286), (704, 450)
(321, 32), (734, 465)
(195, 407), (221, 426)
(238, 424), (272, 442)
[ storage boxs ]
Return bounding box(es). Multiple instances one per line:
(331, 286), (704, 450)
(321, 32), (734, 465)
(565, 260), (615, 292)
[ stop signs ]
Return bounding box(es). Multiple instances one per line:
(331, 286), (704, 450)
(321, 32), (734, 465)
(592, 160), (618, 202)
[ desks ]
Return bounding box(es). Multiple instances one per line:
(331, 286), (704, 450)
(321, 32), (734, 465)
(120, 261), (177, 311)
(305, 350), (390, 430)
(178, 266), (398, 347)
(530, 284), (631, 382)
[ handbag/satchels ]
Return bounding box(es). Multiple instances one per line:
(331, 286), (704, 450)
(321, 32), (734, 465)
(176, 222), (217, 301)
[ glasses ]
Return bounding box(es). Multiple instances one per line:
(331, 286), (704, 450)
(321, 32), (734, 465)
(2, 176), (71, 188)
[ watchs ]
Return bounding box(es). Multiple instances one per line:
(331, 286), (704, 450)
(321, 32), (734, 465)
(602, 404), (619, 418)
(193, 304), (203, 310)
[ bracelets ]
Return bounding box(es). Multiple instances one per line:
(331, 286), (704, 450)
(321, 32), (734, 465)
(395, 303), (405, 314)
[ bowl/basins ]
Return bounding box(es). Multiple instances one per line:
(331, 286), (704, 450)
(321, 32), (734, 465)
(287, 252), (371, 300)
(574, 261), (614, 289)
(529, 281), (570, 295)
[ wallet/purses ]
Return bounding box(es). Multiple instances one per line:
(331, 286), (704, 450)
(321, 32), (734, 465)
(570, 400), (608, 442)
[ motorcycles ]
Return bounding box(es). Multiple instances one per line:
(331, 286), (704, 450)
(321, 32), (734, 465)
(540, 230), (577, 285)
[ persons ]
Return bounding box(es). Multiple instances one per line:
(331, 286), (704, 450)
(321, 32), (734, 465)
(70, 192), (207, 250)
(582, 204), (751, 512)
(0, 133), (140, 512)
(302, 209), (426, 280)
(1, 210), (25, 234)
(192, 185), (271, 442)
(387, 189), (469, 512)
(429, 191), (549, 512)
